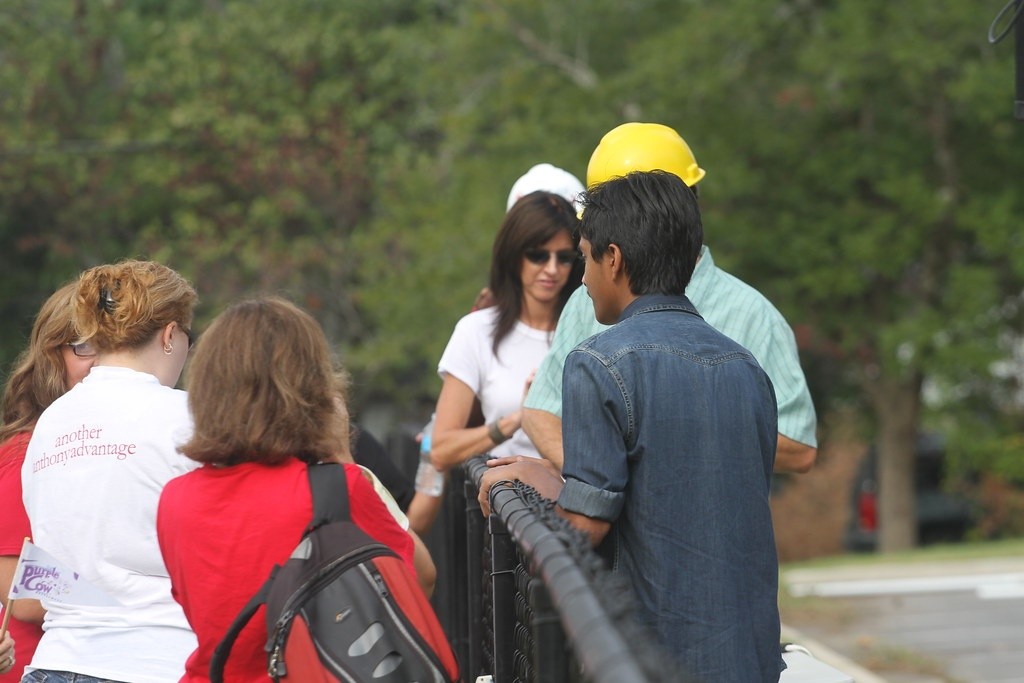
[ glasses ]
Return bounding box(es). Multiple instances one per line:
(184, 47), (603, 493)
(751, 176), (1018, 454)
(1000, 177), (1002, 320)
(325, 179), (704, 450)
(62, 337), (97, 356)
(525, 248), (579, 263)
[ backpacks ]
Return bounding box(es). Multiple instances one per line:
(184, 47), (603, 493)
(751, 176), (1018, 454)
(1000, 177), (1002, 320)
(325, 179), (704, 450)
(208, 462), (463, 683)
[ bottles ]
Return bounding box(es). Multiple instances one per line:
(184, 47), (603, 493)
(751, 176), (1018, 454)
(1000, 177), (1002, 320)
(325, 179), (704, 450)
(414, 412), (446, 497)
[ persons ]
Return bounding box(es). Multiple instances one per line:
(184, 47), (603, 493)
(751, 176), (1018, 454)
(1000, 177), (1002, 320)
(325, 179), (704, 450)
(18, 261), (200, 683)
(519, 122), (818, 472)
(157, 294), (438, 682)
(0, 630), (16, 674)
(0, 281), (100, 683)
(348, 423), (446, 537)
(476, 169), (787, 683)
(430, 192), (584, 461)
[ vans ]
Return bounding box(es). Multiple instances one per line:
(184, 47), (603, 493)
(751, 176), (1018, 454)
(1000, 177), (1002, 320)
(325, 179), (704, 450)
(846, 434), (971, 551)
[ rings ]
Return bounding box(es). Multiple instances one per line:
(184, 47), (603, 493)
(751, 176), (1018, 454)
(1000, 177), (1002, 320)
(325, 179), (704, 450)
(9, 656), (16, 666)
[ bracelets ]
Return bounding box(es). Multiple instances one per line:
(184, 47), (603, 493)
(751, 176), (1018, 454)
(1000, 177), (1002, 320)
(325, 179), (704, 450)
(488, 417), (513, 446)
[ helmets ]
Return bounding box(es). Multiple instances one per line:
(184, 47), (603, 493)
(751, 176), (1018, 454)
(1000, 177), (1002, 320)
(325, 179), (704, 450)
(505, 163), (589, 221)
(587, 122), (708, 200)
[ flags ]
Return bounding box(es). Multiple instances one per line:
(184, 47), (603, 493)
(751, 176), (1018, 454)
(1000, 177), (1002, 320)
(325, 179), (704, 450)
(8, 541), (81, 609)
